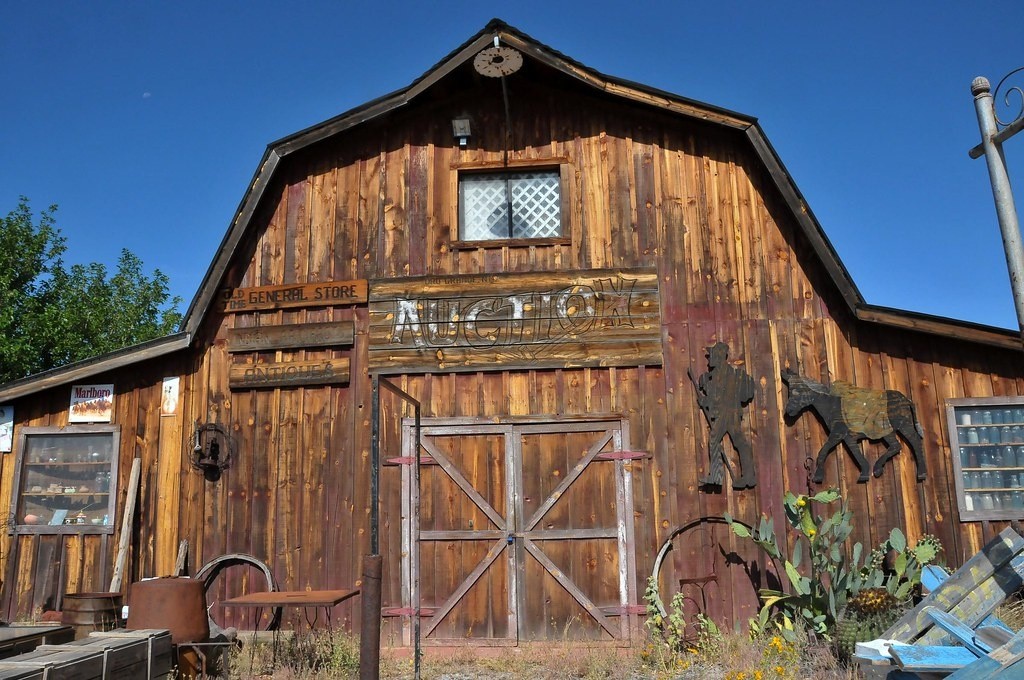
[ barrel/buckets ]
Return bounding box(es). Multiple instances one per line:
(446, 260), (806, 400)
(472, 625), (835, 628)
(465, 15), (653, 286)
(60, 592), (123, 641)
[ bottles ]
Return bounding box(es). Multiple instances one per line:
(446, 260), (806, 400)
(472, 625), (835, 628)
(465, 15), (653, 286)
(960, 409), (1024, 511)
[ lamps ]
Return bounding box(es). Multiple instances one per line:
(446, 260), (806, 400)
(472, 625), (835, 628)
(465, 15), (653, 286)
(452, 118), (472, 148)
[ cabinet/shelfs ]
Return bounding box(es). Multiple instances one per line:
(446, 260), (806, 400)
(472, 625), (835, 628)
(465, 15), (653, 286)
(957, 423), (1024, 492)
(21, 461), (111, 495)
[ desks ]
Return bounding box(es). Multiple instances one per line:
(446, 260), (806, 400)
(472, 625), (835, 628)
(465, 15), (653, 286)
(0, 626), (76, 661)
(222, 590), (360, 676)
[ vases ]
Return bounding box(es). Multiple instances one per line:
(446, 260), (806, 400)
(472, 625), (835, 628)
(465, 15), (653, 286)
(954, 408), (1024, 511)
